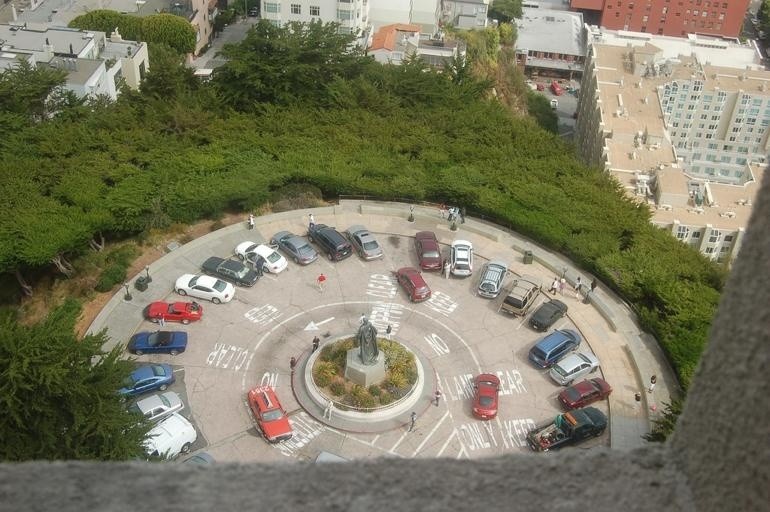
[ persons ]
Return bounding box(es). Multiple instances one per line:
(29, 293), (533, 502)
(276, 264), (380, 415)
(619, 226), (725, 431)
(439, 256), (449, 278)
(357, 311), (365, 326)
(434, 386), (443, 407)
(246, 213), (256, 232)
(317, 272), (327, 293)
(385, 323), (393, 340)
(547, 274), (597, 302)
(312, 335), (321, 354)
(408, 411), (419, 435)
(439, 201), (466, 224)
(289, 356), (297, 375)
(155, 311), (165, 328)
(320, 399), (334, 422)
(444, 262), (453, 280)
(632, 390), (644, 420)
(354, 316), (379, 365)
(308, 211), (317, 227)
(648, 372), (661, 395)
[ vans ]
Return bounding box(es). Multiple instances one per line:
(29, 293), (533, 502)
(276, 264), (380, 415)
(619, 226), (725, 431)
(139, 411), (199, 463)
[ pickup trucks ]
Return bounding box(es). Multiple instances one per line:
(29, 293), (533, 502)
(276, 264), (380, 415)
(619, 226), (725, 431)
(524, 405), (609, 454)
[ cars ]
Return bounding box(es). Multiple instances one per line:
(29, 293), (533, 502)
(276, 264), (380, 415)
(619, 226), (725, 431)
(756, 30), (767, 40)
(750, 18), (759, 26)
(200, 256), (261, 288)
(413, 230), (443, 272)
(120, 390), (186, 435)
(470, 372), (501, 421)
(119, 361), (176, 397)
(173, 272), (236, 304)
(557, 376), (614, 411)
(248, 6), (260, 18)
(536, 81), (563, 111)
(125, 329), (190, 356)
(233, 239), (289, 275)
(246, 384), (294, 444)
(269, 230), (319, 267)
(345, 224), (386, 263)
(310, 450), (346, 462)
(146, 300), (205, 325)
(178, 449), (217, 463)
(528, 298), (569, 332)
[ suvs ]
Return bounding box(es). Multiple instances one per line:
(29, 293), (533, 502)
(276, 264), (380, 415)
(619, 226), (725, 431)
(499, 273), (544, 317)
(395, 266), (432, 304)
(305, 223), (354, 262)
(449, 239), (475, 278)
(548, 350), (600, 387)
(528, 328), (583, 371)
(476, 260), (509, 300)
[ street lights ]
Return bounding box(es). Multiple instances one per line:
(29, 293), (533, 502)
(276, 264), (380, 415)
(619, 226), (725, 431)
(434, 389), (442, 406)
(134, 1), (148, 11)
(408, 410), (417, 433)
(122, 283), (133, 302)
(567, 64), (573, 89)
(407, 205), (416, 222)
(144, 265), (153, 282)
(562, 265), (569, 278)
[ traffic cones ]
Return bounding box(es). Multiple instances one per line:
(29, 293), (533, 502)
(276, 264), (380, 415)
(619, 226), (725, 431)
(650, 403), (658, 411)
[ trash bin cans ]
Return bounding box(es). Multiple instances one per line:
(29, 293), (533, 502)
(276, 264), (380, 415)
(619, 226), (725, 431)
(523, 250), (533, 264)
